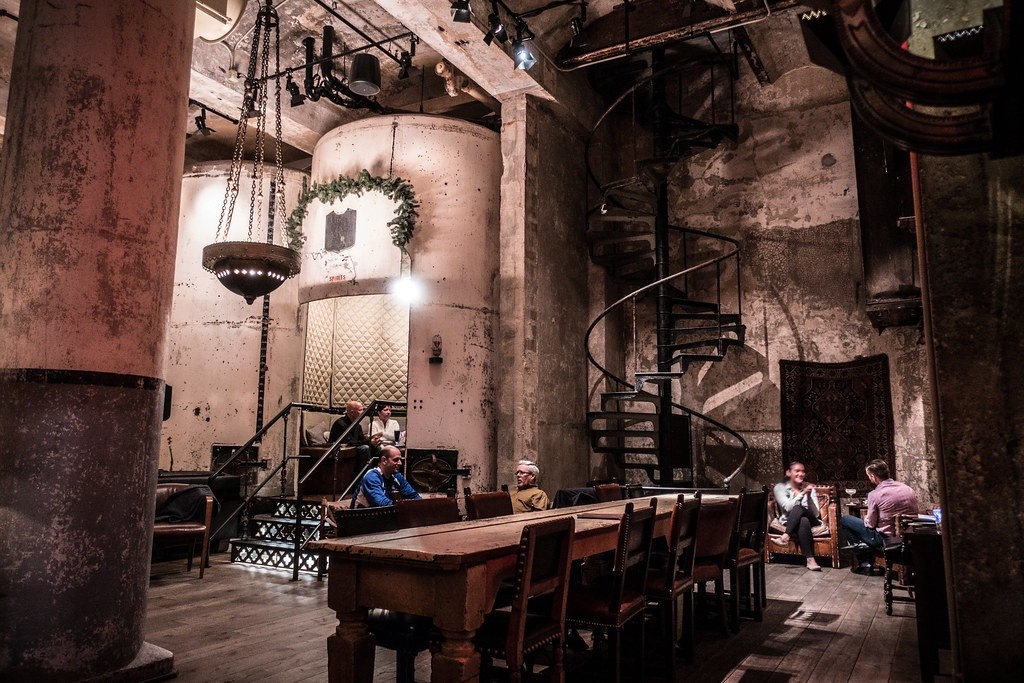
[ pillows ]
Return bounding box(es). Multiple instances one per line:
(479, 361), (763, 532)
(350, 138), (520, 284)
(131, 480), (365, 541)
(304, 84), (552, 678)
(817, 493), (829, 523)
(306, 421), (330, 446)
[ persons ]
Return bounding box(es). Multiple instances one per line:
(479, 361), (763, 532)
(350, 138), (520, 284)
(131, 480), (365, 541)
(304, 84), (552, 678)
(771, 461), (820, 570)
(328, 401), (381, 467)
(508, 460), (549, 514)
(841, 460), (919, 575)
(368, 404), (400, 446)
(354, 445), (422, 509)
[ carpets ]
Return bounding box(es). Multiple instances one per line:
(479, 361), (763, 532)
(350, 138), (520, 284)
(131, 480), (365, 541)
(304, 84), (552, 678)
(779, 354), (897, 499)
(535, 592), (804, 683)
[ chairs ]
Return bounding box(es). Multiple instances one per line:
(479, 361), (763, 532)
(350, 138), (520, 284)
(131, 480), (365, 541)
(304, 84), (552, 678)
(697, 484), (768, 628)
(607, 490), (704, 657)
(332, 506), (439, 683)
(595, 483), (625, 503)
(688, 497), (738, 636)
(464, 484), (514, 521)
(552, 487), (600, 509)
(427, 516), (575, 683)
(625, 483), (644, 498)
(523, 498), (658, 683)
(152, 483), (220, 579)
(398, 497), (514, 610)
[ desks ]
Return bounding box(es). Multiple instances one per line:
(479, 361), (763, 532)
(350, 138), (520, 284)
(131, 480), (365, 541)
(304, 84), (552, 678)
(306, 493), (740, 683)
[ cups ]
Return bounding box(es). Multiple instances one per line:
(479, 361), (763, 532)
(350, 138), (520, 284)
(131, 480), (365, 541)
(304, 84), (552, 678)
(394, 430), (401, 446)
(933, 509), (942, 535)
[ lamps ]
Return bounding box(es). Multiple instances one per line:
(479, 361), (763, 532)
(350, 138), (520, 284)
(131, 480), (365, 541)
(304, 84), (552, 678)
(201, 0), (301, 307)
(448, 0), (476, 24)
(511, 24), (538, 71)
(348, 53), (381, 96)
(429, 333), (444, 364)
(285, 73), (308, 108)
(483, 0), (509, 46)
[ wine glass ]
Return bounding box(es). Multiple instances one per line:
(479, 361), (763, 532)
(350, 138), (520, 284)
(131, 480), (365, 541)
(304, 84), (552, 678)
(845, 488), (856, 505)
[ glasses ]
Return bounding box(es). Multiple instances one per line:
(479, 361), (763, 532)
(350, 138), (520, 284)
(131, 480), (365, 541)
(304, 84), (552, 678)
(515, 471), (532, 476)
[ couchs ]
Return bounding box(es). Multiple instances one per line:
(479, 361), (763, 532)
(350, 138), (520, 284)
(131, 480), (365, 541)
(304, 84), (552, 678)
(869, 502), (941, 585)
(298, 409), (406, 499)
(764, 485), (842, 569)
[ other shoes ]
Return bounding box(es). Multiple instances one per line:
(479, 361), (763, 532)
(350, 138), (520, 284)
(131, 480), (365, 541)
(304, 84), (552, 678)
(771, 538), (789, 548)
(851, 566), (874, 575)
(807, 565), (822, 571)
